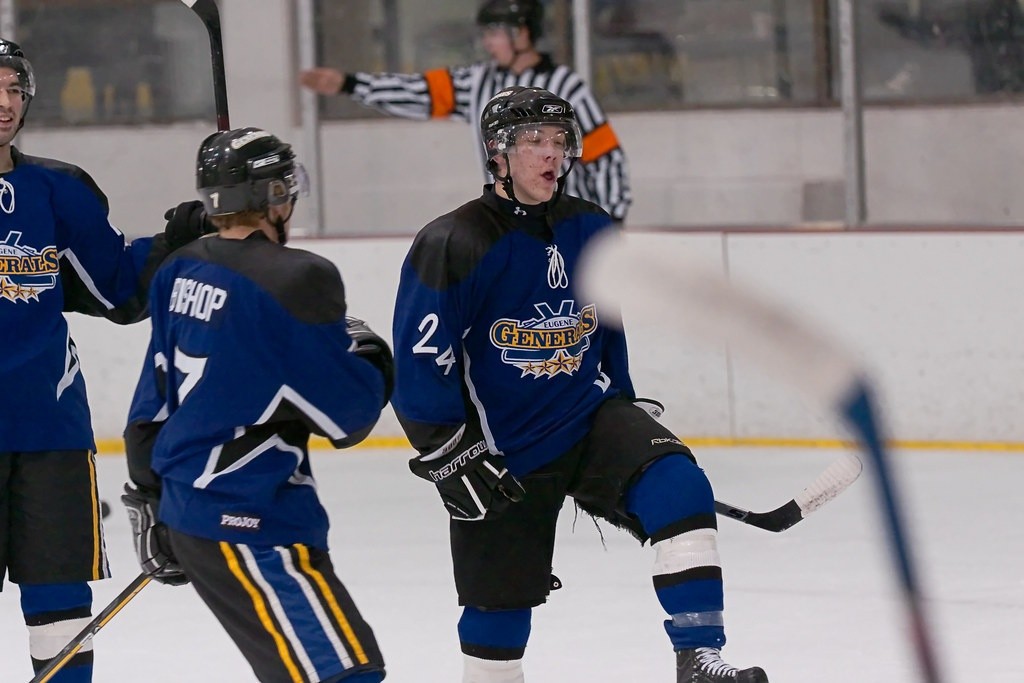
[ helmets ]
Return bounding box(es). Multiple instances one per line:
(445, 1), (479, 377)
(0, 38), (36, 96)
(479, 85), (583, 161)
(194, 126), (310, 216)
(477, 0), (543, 23)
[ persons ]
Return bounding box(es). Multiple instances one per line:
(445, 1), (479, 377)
(123, 126), (393, 683)
(299, 0), (634, 220)
(864, 9), (980, 103)
(395, 87), (767, 683)
(0, 34), (218, 683)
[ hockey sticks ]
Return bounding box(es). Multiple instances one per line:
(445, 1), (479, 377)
(714, 455), (863, 533)
(176, 0), (230, 129)
(571, 221), (950, 683)
(30, 571), (153, 683)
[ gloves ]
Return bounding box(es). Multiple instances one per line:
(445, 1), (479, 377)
(346, 315), (395, 399)
(120, 483), (190, 586)
(164, 199), (208, 249)
(408, 418), (526, 522)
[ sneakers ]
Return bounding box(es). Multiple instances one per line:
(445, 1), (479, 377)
(676, 647), (769, 683)
